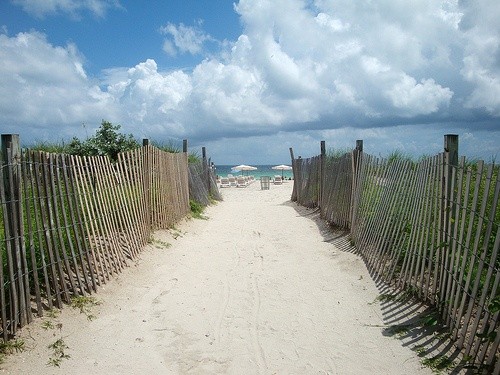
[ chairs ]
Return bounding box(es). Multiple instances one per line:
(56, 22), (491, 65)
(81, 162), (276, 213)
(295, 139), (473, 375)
(220, 175), (255, 187)
(260, 175), (269, 190)
(273, 175), (282, 184)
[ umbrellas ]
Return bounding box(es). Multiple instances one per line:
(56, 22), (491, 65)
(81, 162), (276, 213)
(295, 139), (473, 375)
(232, 164), (293, 181)
